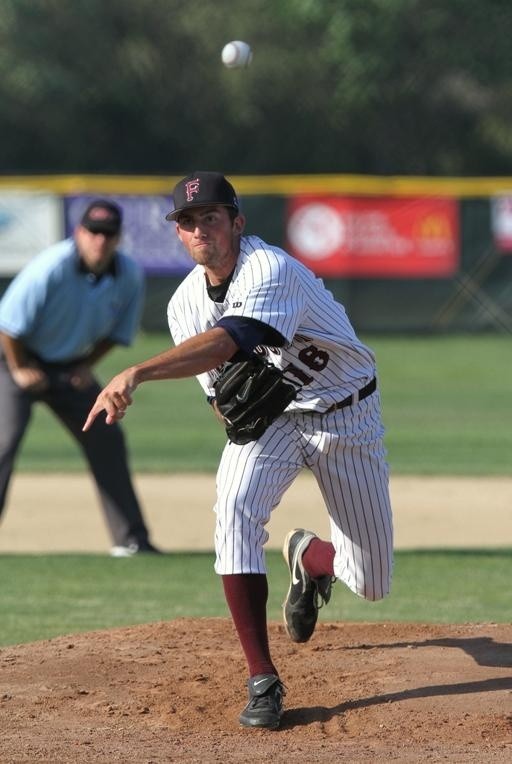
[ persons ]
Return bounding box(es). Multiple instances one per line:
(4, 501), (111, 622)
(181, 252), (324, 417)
(80, 171), (394, 729)
(0, 197), (168, 557)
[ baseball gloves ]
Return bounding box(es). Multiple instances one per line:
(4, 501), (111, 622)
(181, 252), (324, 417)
(206, 351), (302, 445)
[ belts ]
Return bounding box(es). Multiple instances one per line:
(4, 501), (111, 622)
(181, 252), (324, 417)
(327, 376), (376, 413)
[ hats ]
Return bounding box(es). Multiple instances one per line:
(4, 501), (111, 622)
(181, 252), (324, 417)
(165, 172), (238, 221)
(81, 199), (121, 234)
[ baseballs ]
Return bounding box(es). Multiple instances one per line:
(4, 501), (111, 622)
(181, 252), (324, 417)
(220, 40), (255, 69)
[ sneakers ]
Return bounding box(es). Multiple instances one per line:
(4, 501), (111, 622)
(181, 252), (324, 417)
(283, 527), (333, 642)
(110, 539), (159, 557)
(238, 673), (283, 728)
